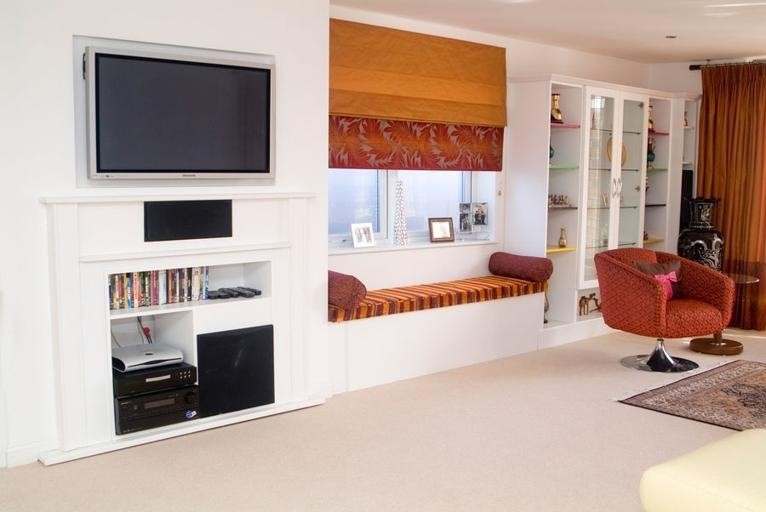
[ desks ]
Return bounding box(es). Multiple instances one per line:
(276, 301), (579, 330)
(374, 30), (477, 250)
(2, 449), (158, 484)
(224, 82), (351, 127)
(690, 273), (760, 355)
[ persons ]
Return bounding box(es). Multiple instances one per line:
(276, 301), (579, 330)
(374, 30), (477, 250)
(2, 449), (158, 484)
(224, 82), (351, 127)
(460, 205), (486, 232)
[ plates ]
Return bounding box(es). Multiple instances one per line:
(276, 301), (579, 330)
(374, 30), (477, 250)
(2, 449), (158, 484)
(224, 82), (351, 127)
(607, 138), (627, 168)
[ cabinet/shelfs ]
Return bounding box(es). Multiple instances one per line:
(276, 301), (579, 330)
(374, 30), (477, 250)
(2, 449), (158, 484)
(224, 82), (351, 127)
(504, 72), (702, 349)
(78, 242), (294, 459)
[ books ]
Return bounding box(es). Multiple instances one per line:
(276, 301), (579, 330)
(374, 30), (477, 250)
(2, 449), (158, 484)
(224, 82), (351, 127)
(107, 265), (210, 310)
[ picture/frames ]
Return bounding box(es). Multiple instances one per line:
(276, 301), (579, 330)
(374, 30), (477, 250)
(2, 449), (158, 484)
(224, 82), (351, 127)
(428, 218), (454, 242)
(351, 223), (376, 248)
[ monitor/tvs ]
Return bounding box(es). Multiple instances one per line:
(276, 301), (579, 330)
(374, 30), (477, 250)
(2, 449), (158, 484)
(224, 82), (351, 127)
(84, 45), (277, 180)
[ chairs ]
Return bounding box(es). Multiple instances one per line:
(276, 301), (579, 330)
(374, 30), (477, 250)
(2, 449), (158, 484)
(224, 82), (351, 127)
(594, 248), (736, 372)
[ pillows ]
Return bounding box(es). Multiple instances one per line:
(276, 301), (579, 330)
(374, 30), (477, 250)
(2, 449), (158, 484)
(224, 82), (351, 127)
(637, 259), (684, 299)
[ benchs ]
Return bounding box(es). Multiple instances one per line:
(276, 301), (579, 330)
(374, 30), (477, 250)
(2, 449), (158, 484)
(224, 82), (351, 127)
(329, 274), (548, 398)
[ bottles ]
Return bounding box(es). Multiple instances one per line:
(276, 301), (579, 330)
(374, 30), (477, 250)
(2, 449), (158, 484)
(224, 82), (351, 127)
(559, 228), (567, 247)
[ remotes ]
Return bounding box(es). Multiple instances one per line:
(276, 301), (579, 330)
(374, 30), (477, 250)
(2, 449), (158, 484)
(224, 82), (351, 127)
(230, 288), (255, 298)
(216, 291), (230, 298)
(218, 288), (240, 297)
(237, 287), (261, 295)
(208, 292), (218, 299)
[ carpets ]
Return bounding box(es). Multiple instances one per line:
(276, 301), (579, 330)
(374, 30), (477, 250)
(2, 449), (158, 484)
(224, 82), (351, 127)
(617, 360), (766, 431)
